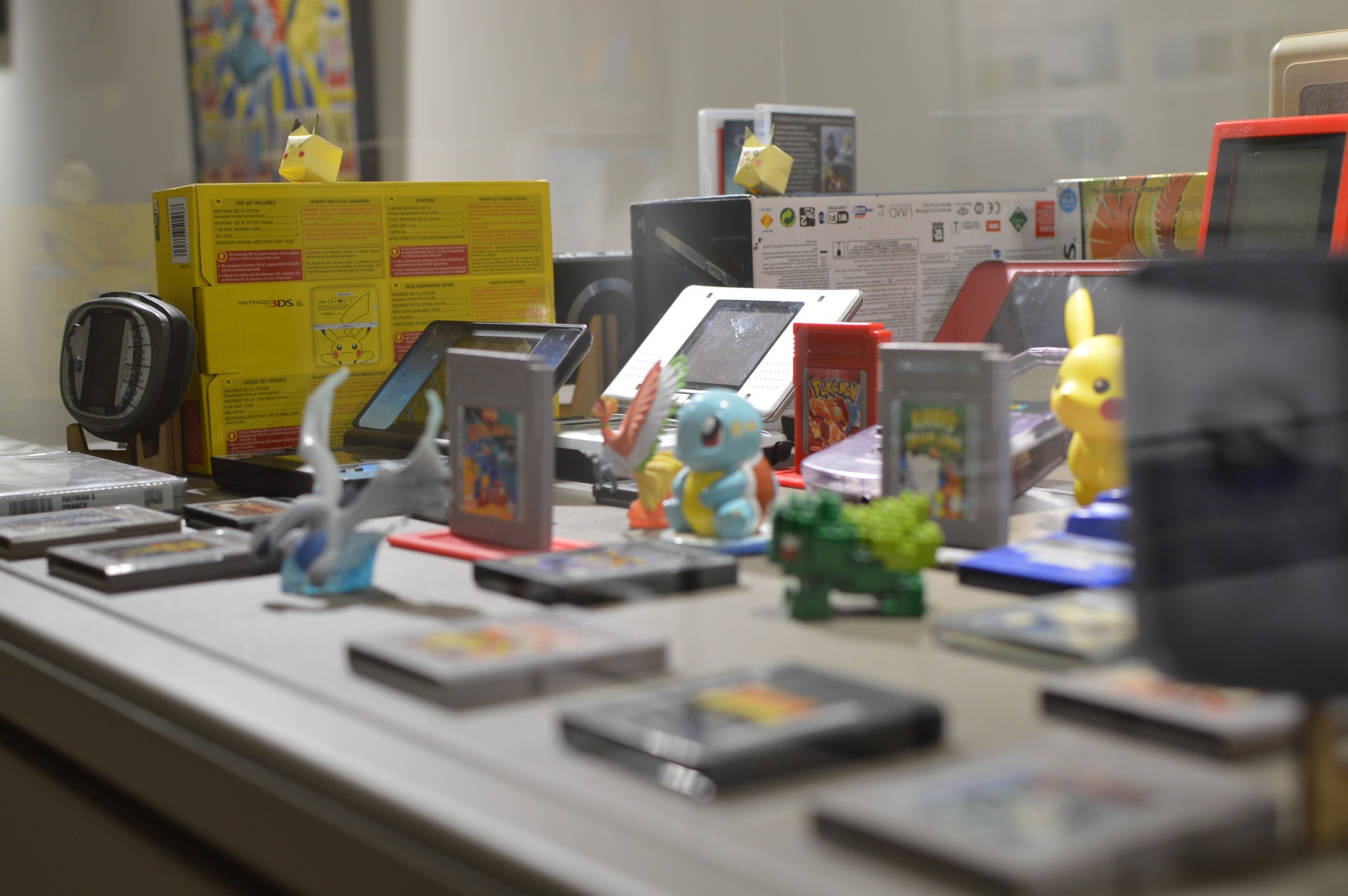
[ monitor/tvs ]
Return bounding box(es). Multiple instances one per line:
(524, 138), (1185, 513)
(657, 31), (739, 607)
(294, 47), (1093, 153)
(1196, 114), (1347, 263)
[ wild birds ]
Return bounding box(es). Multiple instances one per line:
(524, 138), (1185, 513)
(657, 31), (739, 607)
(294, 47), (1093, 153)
(246, 368), (451, 584)
(590, 355), (689, 494)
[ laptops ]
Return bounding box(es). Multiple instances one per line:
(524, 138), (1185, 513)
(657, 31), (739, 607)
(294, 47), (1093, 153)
(928, 257), (1153, 359)
(213, 321), (593, 504)
(558, 285), (862, 461)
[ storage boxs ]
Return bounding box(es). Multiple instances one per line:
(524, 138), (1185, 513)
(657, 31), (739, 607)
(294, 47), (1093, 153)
(153, 170), (1210, 491)
